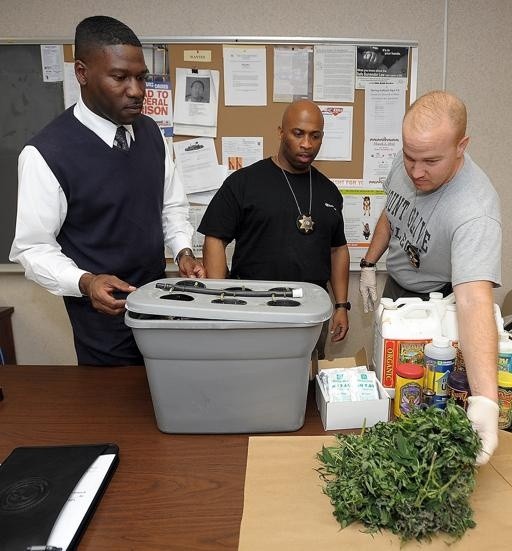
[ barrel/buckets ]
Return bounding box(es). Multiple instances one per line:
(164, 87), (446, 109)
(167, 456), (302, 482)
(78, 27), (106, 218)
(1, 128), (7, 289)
(370, 292), (504, 399)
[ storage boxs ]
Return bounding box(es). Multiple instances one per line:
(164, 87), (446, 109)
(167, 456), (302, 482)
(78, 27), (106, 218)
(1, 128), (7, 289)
(123, 276), (334, 436)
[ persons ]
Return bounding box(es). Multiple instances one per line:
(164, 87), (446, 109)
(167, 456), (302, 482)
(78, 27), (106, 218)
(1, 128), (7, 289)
(197, 99), (353, 358)
(357, 46), (383, 71)
(359, 92), (502, 468)
(12, 16), (206, 367)
(187, 81), (208, 102)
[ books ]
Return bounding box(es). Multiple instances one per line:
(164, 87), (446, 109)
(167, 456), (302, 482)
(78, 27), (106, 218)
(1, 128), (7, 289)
(1, 444), (122, 551)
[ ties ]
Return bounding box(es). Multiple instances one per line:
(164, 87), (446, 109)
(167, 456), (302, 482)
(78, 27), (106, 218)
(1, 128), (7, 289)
(115, 127), (130, 151)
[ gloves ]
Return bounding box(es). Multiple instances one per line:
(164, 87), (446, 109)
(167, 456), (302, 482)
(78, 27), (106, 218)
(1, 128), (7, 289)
(360, 268), (377, 314)
(466, 395), (500, 464)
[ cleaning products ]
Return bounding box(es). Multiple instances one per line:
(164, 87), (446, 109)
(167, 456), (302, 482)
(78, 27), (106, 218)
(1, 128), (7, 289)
(418, 336), (457, 407)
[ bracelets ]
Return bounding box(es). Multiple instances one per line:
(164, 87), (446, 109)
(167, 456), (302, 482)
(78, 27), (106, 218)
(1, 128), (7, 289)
(335, 302), (351, 310)
(177, 248), (194, 261)
(360, 258), (375, 268)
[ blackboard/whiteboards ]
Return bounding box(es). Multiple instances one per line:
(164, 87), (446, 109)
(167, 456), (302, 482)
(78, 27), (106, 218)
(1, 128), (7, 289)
(0, 44), (65, 265)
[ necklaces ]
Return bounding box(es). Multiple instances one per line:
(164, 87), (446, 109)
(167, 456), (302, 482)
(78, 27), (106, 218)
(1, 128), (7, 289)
(407, 183), (449, 269)
(275, 158), (317, 234)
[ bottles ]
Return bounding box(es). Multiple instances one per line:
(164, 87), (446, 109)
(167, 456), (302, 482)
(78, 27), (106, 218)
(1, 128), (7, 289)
(393, 363), (425, 420)
(422, 335), (458, 406)
(494, 342), (512, 374)
(448, 372), (470, 416)
(494, 373), (512, 433)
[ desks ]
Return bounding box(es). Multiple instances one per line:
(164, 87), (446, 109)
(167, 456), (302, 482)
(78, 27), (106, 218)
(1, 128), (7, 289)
(0, 306), (17, 365)
(0, 364), (512, 551)
